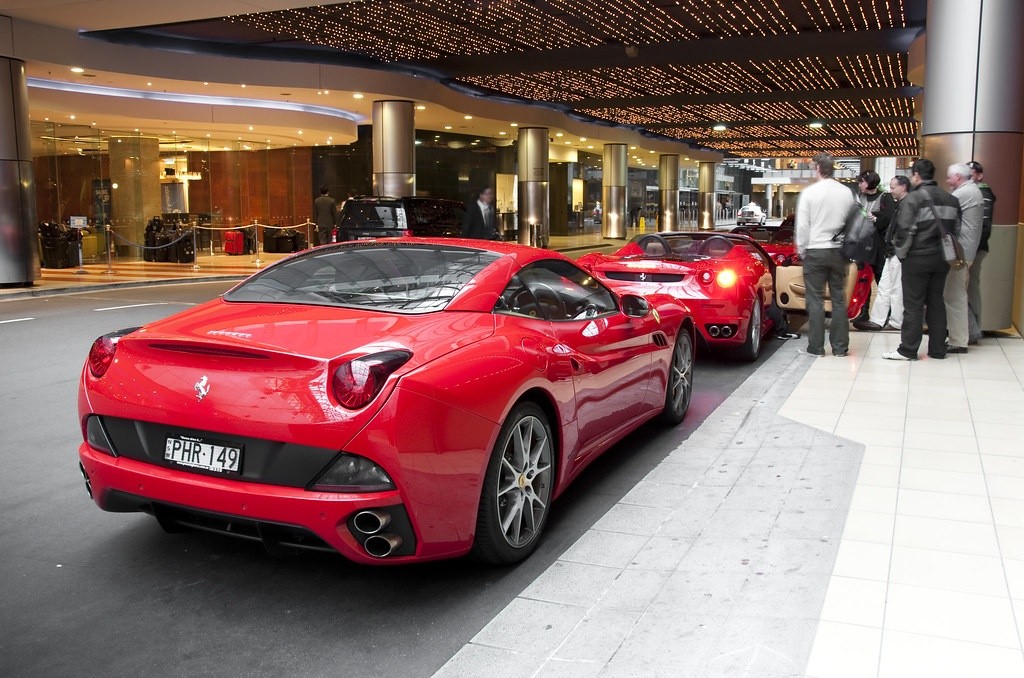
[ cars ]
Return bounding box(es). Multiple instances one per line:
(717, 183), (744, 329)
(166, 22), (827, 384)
(729, 225), (797, 265)
(737, 202), (767, 225)
(780, 215), (794, 230)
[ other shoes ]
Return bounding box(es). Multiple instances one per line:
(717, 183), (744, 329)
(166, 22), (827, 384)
(776, 333), (800, 340)
(853, 321), (881, 330)
(883, 323), (899, 331)
(946, 342), (967, 353)
(798, 348), (825, 357)
(881, 350), (916, 361)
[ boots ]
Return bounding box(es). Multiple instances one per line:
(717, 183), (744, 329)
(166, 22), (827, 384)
(850, 306), (869, 322)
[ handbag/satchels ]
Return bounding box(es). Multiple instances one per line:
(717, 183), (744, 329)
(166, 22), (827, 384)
(942, 232), (965, 270)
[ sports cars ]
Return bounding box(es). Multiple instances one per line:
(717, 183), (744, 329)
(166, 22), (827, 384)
(558, 230), (875, 363)
(78, 237), (699, 573)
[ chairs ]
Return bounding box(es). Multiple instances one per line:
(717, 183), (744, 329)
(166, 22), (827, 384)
(699, 235), (734, 254)
(638, 233), (672, 258)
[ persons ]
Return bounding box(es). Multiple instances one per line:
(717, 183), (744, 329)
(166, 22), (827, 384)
(794, 153), (856, 358)
(882, 158), (962, 361)
(853, 174), (912, 330)
(967, 160), (998, 346)
(313, 186), (338, 244)
(342, 189), (359, 241)
(844, 170), (897, 329)
(943, 161), (986, 356)
(461, 181), (497, 240)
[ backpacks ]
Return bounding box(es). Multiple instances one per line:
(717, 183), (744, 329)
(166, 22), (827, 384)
(833, 188), (877, 265)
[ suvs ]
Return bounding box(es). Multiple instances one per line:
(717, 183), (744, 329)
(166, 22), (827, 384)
(330, 194), (488, 246)
